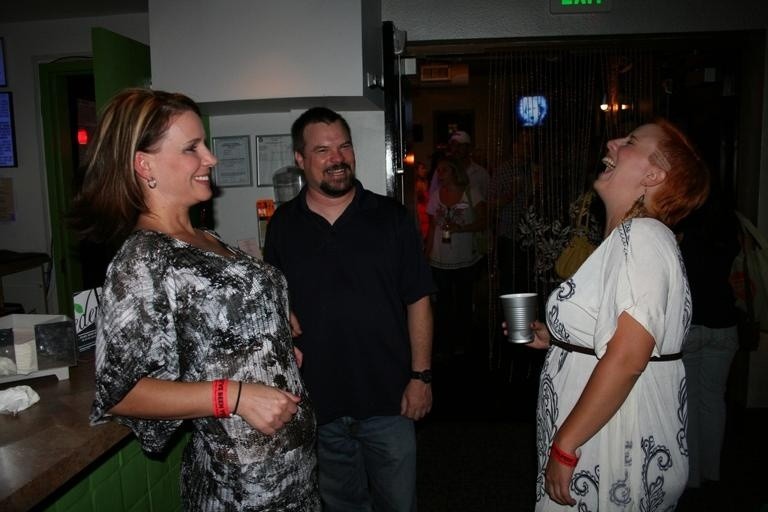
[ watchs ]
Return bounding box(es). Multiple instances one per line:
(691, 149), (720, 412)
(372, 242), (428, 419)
(411, 368), (432, 384)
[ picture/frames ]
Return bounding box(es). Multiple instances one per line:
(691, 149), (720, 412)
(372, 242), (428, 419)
(212, 133), (297, 188)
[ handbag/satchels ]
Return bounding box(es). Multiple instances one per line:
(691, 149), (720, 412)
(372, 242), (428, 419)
(726, 204), (768, 333)
(554, 221), (601, 281)
(474, 232), (497, 255)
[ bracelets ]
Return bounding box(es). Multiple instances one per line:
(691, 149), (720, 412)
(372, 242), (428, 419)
(212, 378), (230, 419)
(550, 442), (579, 468)
(233, 380), (242, 415)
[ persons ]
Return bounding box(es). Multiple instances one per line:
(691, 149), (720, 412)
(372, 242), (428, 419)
(417, 157), (430, 203)
(261, 107), (437, 510)
(671, 179), (755, 504)
(425, 151), (493, 361)
(76, 90), (327, 512)
(432, 130), (487, 205)
(498, 116), (709, 510)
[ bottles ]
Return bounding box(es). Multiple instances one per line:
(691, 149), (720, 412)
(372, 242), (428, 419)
(441, 208), (452, 243)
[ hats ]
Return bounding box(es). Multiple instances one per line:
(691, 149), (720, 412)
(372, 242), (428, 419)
(450, 129), (472, 145)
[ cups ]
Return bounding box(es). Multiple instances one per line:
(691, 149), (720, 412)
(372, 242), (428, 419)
(502, 292), (537, 343)
